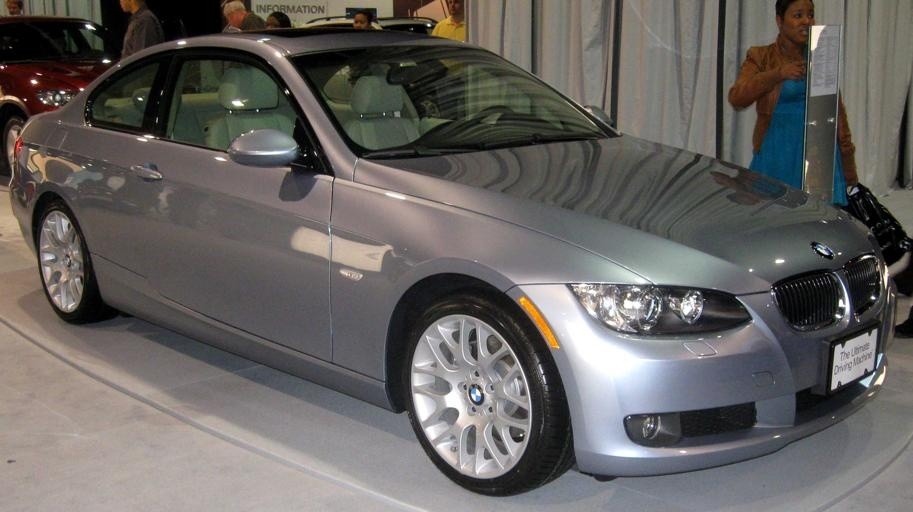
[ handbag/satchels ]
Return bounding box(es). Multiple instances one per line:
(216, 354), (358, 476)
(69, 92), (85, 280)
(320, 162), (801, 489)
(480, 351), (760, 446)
(845, 182), (913, 267)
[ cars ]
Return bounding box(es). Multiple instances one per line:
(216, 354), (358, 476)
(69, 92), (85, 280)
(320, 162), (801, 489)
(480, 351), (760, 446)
(0, 11), (205, 182)
(4, 23), (900, 503)
(293, 9), (444, 114)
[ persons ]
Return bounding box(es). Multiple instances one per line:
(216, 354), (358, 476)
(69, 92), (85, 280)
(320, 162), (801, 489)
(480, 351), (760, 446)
(347, 8), (374, 89)
(429, 0), (469, 121)
(888, 248), (913, 339)
(728, 0), (859, 210)
(222, 0), (264, 31)
(263, 11), (291, 29)
(3, 0), (24, 14)
(119, 0), (164, 96)
(709, 160), (809, 211)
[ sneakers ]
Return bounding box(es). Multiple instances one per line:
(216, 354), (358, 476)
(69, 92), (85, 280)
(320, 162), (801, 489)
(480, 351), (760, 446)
(893, 304), (913, 338)
